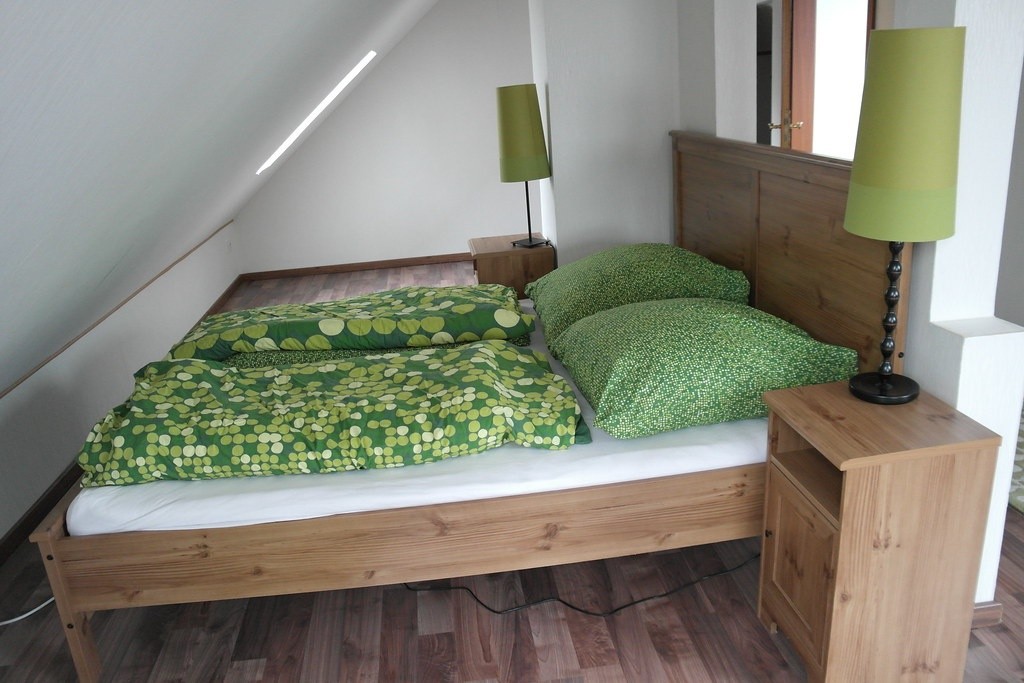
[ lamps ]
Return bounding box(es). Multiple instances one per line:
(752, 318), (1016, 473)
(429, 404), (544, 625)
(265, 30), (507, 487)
(494, 83), (553, 249)
(844, 25), (969, 403)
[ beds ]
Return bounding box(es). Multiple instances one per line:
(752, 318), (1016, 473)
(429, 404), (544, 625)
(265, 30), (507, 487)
(25, 125), (913, 683)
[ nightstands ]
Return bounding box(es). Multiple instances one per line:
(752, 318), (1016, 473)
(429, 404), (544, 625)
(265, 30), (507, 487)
(754, 374), (1003, 683)
(466, 231), (558, 297)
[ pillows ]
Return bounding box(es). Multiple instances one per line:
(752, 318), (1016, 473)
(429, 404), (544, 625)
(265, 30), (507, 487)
(548, 298), (858, 441)
(524, 243), (751, 353)
